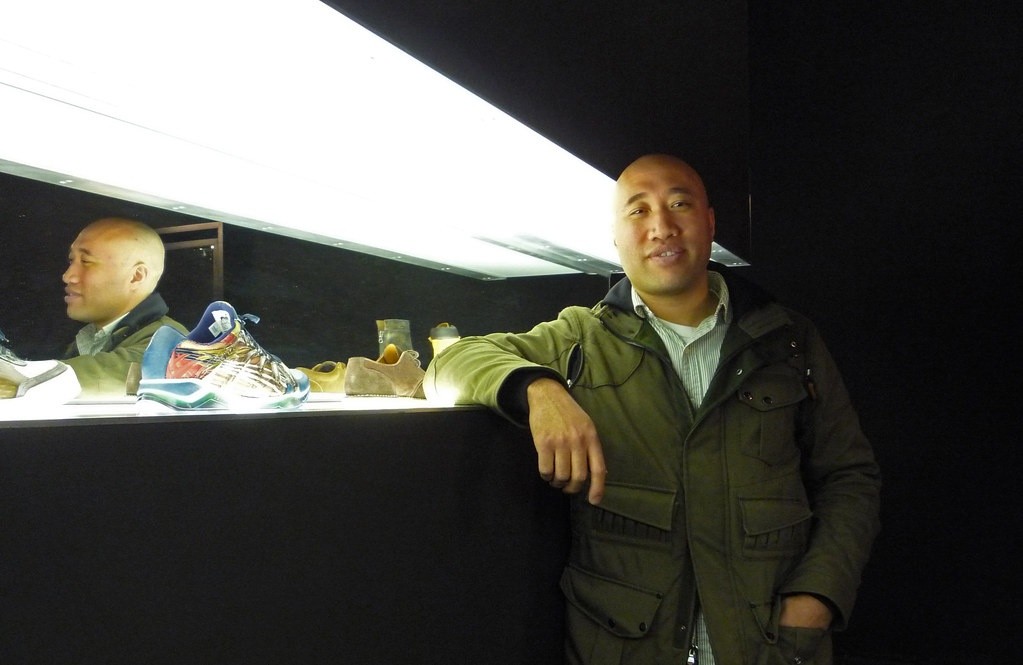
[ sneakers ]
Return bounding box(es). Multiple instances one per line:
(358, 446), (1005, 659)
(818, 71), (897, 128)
(1, 344), (81, 409)
(137, 300), (309, 416)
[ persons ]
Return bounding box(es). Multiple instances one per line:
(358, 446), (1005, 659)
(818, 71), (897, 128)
(422, 154), (888, 664)
(46, 218), (193, 392)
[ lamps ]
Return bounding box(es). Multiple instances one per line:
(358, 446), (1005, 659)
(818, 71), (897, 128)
(2, 0), (754, 285)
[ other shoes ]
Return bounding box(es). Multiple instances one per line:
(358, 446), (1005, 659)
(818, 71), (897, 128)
(292, 360), (345, 402)
(376, 318), (413, 358)
(428, 322), (461, 360)
(342, 344), (426, 404)
